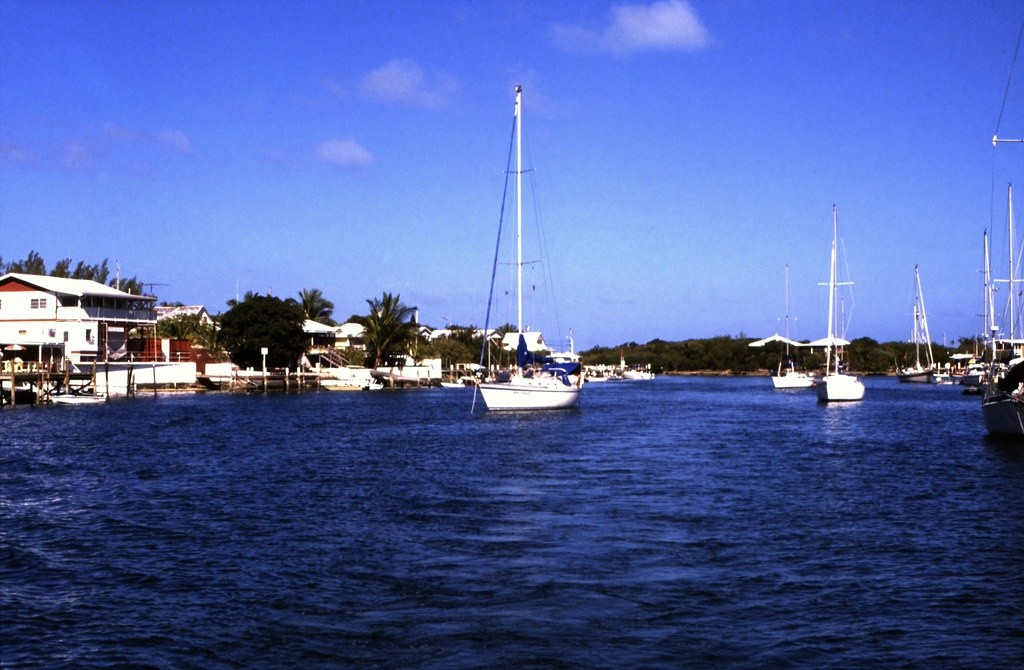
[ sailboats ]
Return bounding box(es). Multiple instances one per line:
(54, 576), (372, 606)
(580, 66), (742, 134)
(464, 83), (582, 413)
(898, 182), (1024, 440)
(748, 205), (868, 402)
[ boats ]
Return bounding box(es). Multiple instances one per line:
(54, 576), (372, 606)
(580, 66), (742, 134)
(50, 393), (108, 406)
(319, 378), (384, 392)
(442, 381), (466, 389)
(577, 348), (656, 384)
